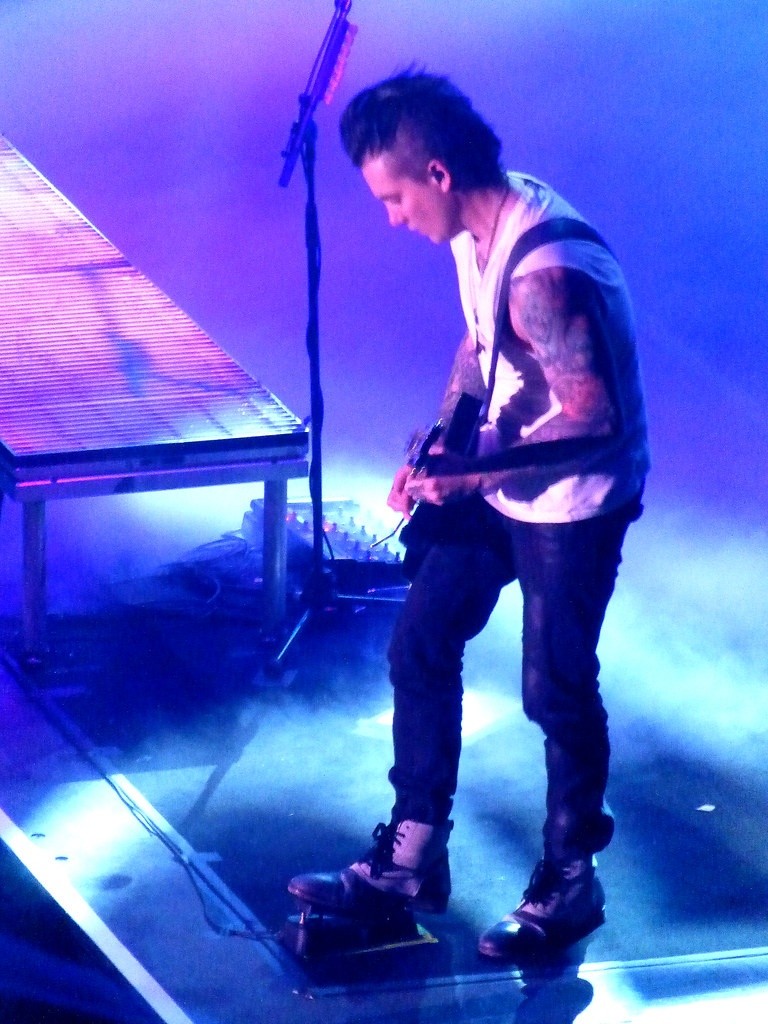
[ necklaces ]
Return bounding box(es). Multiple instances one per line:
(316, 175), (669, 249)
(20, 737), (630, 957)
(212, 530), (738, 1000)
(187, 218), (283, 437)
(480, 183), (510, 275)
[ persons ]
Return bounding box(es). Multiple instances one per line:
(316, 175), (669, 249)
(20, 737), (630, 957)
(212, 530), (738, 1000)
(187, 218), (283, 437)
(288, 73), (651, 957)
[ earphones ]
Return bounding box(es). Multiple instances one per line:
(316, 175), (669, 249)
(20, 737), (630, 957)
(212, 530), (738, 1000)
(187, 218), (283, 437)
(431, 167), (444, 183)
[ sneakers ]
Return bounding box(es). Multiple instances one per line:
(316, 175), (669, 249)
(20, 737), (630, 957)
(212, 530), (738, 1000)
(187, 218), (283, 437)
(287, 807), (453, 916)
(477, 857), (609, 956)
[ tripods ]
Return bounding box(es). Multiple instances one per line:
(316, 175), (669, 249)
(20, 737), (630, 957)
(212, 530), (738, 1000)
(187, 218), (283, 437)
(186, 1), (405, 675)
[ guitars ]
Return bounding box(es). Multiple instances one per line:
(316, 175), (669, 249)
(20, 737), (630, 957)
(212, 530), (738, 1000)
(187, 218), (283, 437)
(400, 391), (485, 584)
